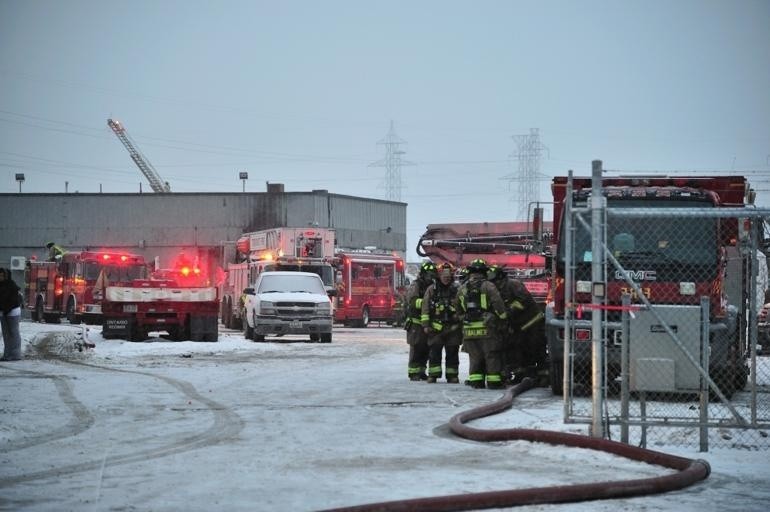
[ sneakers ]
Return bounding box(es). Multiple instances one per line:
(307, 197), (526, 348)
(426, 375), (437, 383)
(446, 377), (459, 383)
(408, 372), (428, 382)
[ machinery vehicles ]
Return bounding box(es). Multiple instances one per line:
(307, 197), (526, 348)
(103, 268), (221, 342)
(223, 226), (336, 330)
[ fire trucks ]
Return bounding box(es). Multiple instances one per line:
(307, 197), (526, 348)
(23, 248), (145, 324)
(333, 247), (411, 329)
(523, 172), (754, 400)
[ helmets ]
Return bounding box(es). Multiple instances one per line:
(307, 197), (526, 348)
(420, 260), (458, 274)
(460, 257), (499, 275)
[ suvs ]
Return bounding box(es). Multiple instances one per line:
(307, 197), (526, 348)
(242, 270), (337, 344)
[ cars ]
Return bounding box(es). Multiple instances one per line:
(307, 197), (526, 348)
(756, 303), (770, 353)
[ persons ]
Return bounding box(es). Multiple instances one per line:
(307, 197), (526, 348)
(419, 263), (466, 385)
(0, 268), (23, 362)
(46, 241), (65, 261)
(485, 265), (552, 391)
(405, 260), (437, 380)
(456, 258), (511, 394)
